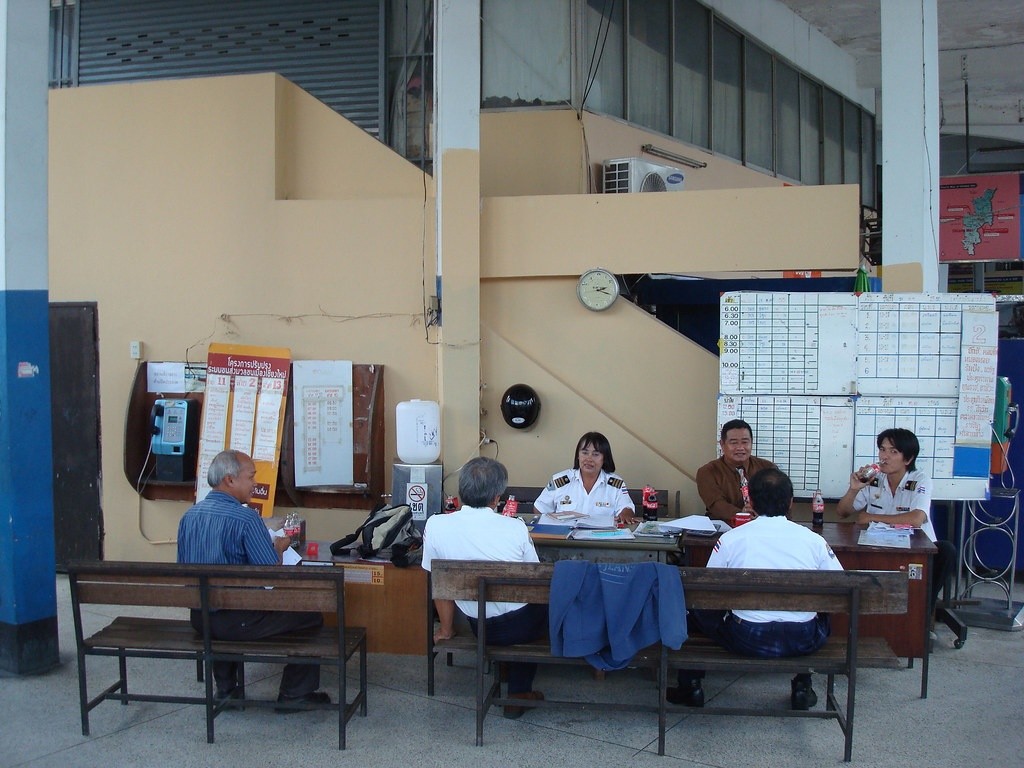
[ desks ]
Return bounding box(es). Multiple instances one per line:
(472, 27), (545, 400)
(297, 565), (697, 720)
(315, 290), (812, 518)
(951, 488), (1024, 631)
(294, 540), (452, 696)
(679, 519), (938, 698)
(498, 512), (682, 563)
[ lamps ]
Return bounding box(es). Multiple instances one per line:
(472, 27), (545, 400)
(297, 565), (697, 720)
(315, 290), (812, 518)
(640, 144), (707, 168)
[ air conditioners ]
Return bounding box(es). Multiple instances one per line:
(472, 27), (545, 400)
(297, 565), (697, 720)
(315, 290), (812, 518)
(603, 157), (685, 194)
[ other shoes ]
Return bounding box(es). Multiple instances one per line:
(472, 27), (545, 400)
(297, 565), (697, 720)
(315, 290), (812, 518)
(214, 690), (245, 710)
(275, 692), (331, 713)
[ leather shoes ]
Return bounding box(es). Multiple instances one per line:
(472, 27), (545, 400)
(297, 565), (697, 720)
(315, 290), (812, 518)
(503, 689), (544, 719)
(666, 679), (704, 707)
(791, 679), (817, 710)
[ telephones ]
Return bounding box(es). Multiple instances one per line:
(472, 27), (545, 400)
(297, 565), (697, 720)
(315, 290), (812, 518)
(149, 398), (196, 455)
(992, 376), (1019, 474)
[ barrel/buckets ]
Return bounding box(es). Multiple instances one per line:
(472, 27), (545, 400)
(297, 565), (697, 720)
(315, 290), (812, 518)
(396, 398), (441, 465)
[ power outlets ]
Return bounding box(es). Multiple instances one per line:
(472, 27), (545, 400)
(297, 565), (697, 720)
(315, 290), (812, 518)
(480, 438), (485, 449)
(131, 341), (142, 359)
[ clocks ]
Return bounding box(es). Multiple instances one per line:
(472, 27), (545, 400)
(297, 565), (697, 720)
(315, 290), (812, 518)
(576, 267), (619, 311)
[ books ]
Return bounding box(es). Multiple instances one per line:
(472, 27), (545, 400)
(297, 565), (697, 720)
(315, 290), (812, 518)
(526, 524), (573, 539)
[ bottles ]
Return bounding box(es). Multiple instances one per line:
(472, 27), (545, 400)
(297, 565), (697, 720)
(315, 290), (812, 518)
(444, 494), (517, 516)
(858, 459), (885, 483)
(284, 511), (301, 547)
(643, 483), (658, 522)
(812, 489), (824, 527)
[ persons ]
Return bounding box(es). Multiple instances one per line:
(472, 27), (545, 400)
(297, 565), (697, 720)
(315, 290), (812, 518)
(696, 420), (779, 520)
(179, 449), (331, 711)
(665, 467), (844, 711)
(421, 457), (544, 718)
(534, 432), (635, 525)
(837, 428), (956, 617)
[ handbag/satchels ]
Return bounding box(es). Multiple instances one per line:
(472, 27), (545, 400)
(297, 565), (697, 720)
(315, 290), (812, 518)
(330, 502), (423, 568)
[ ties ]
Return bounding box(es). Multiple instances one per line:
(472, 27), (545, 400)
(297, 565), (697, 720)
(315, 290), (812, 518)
(736, 468), (750, 507)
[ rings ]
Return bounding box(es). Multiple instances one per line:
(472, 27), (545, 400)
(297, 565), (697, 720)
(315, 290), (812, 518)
(631, 518), (633, 519)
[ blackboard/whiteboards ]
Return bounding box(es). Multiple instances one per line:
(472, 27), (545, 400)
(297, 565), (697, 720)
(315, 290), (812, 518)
(715, 291), (997, 501)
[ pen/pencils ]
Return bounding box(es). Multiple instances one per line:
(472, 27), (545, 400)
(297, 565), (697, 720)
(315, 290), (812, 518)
(530, 516), (538, 525)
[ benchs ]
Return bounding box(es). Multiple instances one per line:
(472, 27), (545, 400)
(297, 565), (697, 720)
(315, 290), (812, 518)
(497, 487), (669, 518)
(67, 561), (368, 750)
(432, 559), (910, 762)
